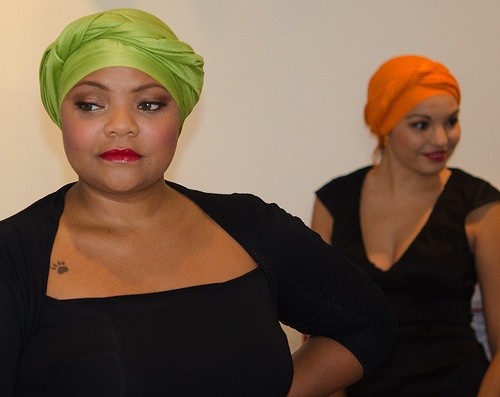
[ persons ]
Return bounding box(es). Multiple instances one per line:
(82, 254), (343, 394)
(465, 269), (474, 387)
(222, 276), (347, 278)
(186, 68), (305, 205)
(1, 8), (397, 397)
(301, 54), (500, 397)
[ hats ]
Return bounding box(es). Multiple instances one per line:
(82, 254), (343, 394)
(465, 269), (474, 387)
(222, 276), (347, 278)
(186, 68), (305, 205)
(364, 56), (462, 147)
(39, 9), (204, 128)
